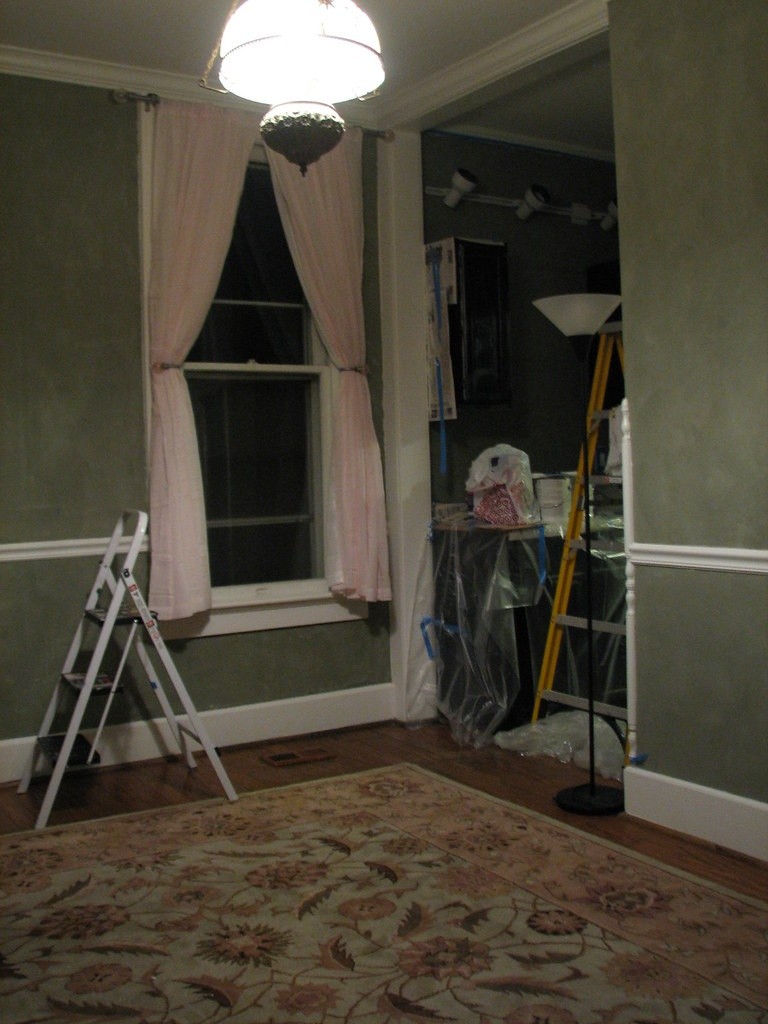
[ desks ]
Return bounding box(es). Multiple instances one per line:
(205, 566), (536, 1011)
(434, 512), (624, 713)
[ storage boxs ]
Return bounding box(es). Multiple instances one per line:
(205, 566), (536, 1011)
(533, 477), (572, 524)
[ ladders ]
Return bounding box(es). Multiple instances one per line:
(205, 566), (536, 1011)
(14, 507), (243, 832)
(528, 320), (634, 768)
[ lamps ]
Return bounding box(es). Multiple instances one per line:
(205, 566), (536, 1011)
(598, 199), (619, 231)
(441, 168), (477, 209)
(516, 184), (551, 219)
(532, 292), (625, 817)
(198, 0), (387, 177)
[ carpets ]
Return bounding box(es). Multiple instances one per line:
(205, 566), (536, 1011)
(0, 761), (768, 1024)
